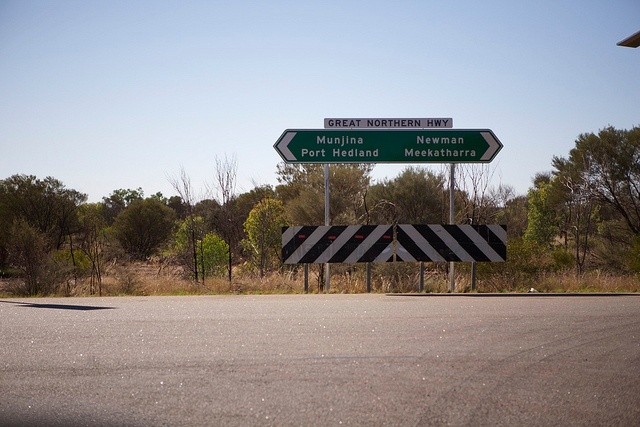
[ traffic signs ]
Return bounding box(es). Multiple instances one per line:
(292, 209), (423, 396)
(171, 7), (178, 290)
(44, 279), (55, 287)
(272, 116), (503, 164)
